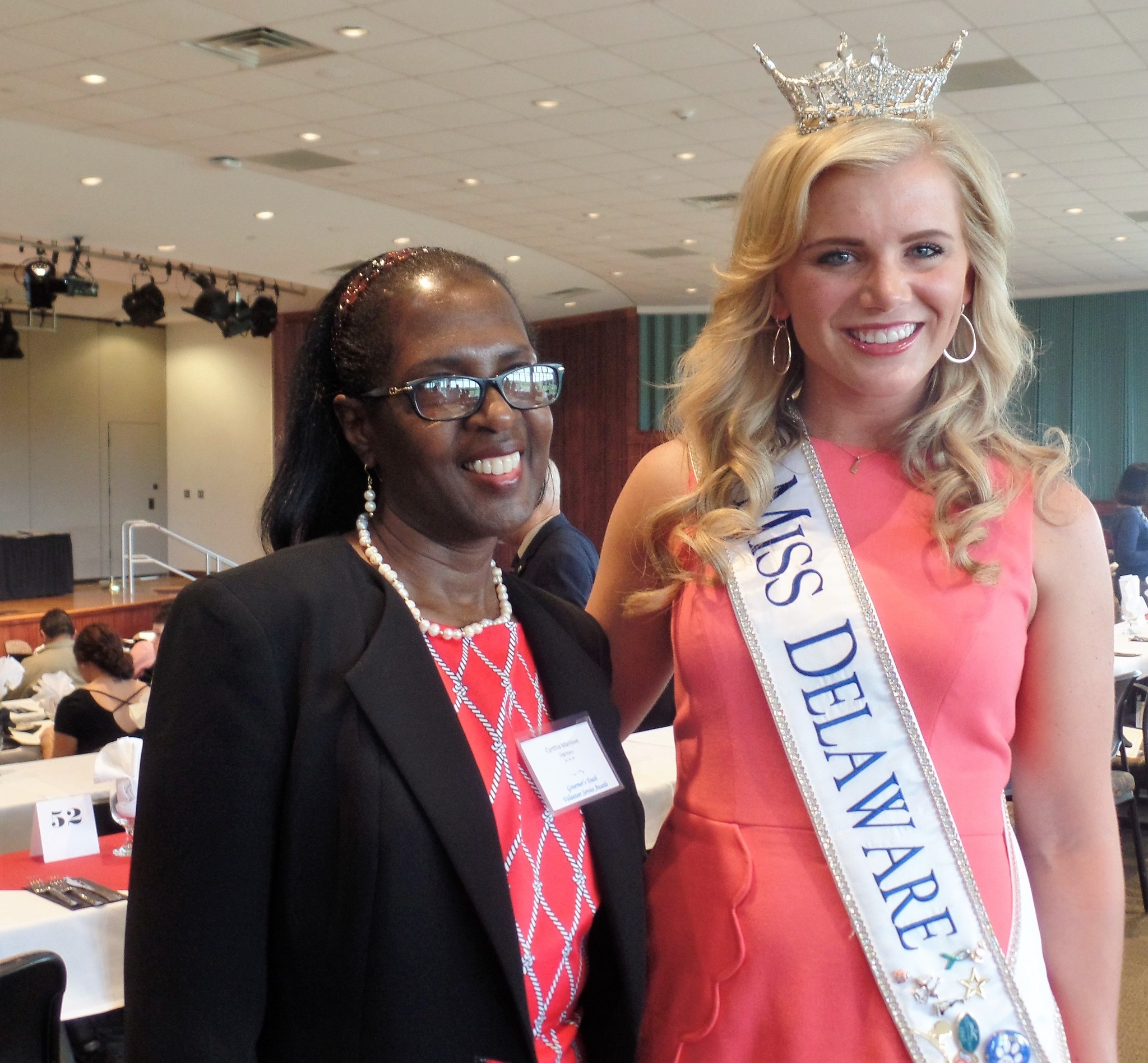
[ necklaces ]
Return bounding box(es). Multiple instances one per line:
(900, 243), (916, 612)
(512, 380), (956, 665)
(355, 512), (512, 641)
(806, 426), (883, 473)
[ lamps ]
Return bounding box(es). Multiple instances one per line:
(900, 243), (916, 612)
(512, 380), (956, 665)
(0, 236), (280, 358)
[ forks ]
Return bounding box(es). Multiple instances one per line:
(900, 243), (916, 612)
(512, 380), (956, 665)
(49, 875), (103, 907)
(26, 874), (78, 908)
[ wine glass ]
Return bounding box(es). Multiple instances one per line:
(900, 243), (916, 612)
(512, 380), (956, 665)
(109, 777), (138, 857)
(1120, 596), (1140, 636)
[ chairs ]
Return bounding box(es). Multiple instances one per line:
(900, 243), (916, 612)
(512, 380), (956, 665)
(1111, 670), (1148, 912)
(0, 949), (66, 1063)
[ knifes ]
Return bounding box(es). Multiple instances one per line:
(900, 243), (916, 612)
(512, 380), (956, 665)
(1115, 653), (1140, 658)
(63, 876), (122, 902)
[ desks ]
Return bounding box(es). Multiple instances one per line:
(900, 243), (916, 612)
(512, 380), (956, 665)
(0, 738), (130, 1023)
(1114, 619), (1148, 679)
(622, 726), (678, 849)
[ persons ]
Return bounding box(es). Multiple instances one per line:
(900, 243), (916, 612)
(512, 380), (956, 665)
(126, 247), (649, 1063)
(1111, 463), (1148, 604)
(584, 29), (1124, 1063)
(499, 457), (600, 609)
(0, 604), (170, 759)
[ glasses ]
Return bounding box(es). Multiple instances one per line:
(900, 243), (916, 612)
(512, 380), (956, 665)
(351, 362), (565, 421)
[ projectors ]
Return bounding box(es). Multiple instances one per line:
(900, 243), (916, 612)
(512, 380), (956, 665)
(55, 278), (99, 297)
